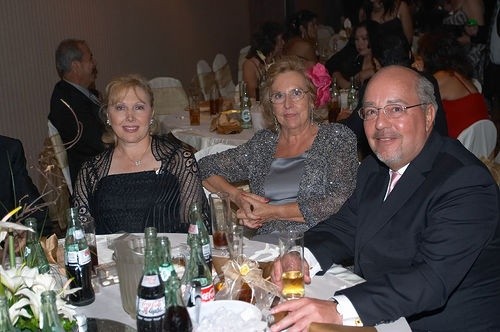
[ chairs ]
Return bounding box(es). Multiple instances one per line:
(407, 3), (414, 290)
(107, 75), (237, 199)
(48, 16), (500, 206)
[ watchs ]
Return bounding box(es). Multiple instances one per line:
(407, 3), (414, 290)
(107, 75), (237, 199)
(333, 300), (343, 316)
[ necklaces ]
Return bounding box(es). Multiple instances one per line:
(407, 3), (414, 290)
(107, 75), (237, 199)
(116, 138), (150, 165)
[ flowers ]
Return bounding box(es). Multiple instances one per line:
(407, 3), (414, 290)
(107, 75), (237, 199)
(0, 206), (83, 332)
(304, 63), (333, 108)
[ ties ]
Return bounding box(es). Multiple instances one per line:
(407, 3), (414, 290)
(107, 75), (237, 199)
(388, 171), (402, 195)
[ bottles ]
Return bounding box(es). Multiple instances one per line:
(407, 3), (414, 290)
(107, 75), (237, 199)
(136, 226), (192, 332)
(64, 208), (95, 305)
(238, 82), (252, 129)
(186, 202), (212, 277)
(330, 78), (341, 112)
(41, 290), (65, 332)
(181, 237), (215, 304)
(23, 218), (50, 275)
(0, 295), (20, 332)
(213, 226), (252, 303)
(347, 76), (358, 110)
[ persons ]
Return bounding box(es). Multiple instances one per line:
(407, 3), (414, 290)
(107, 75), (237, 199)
(0, 134), (50, 252)
(270, 65), (500, 332)
(197, 56), (359, 235)
(74, 75), (211, 234)
(479, 155), (500, 186)
(242, 0), (500, 159)
(48, 39), (114, 188)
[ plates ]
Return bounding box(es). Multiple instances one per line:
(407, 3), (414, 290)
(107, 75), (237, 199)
(185, 299), (262, 332)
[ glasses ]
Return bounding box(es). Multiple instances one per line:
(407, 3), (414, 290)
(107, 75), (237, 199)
(268, 86), (312, 104)
(356, 101), (433, 121)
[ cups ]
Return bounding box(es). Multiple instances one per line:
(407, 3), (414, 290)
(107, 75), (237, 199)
(327, 101), (338, 124)
(209, 88), (219, 118)
(209, 192), (234, 251)
(77, 216), (99, 278)
(188, 95), (201, 127)
(277, 230), (305, 301)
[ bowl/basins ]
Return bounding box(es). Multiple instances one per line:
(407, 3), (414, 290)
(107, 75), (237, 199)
(339, 89), (349, 109)
(250, 110), (265, 132)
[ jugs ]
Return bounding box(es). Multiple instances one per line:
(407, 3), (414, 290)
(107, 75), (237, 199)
(112, 237), (146, 317)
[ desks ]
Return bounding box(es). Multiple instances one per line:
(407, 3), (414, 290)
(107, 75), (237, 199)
(0, 232), (412, 332)
(171, 100), (255, 150)
